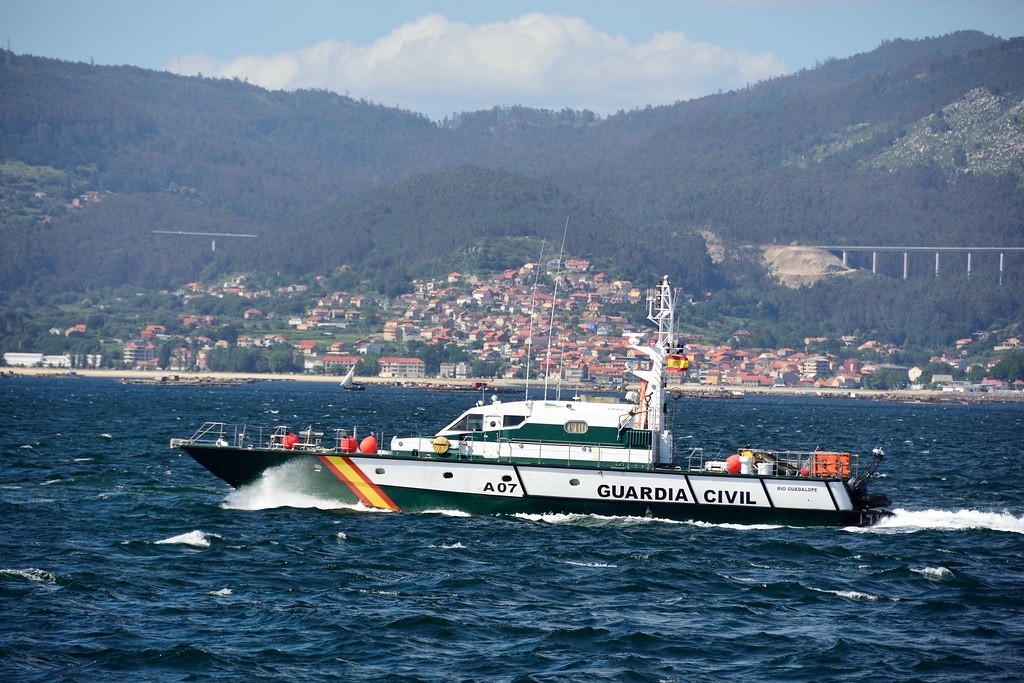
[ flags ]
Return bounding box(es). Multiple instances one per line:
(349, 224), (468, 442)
(668, 355), (688, 371)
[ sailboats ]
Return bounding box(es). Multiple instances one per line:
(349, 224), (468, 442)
(338, 365), (366, 391)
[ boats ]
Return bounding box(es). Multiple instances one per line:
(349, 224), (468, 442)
(169, 217), (893, 527)
